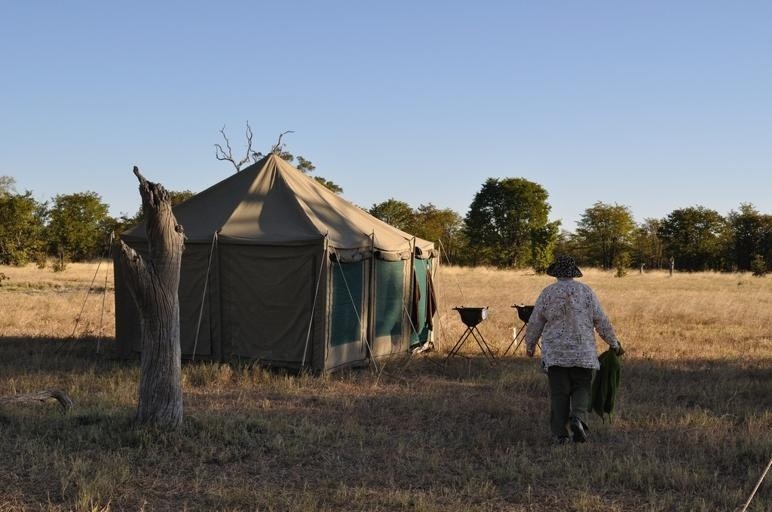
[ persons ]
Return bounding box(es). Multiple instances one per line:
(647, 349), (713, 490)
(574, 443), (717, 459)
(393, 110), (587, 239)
(525, 253), (623, 444)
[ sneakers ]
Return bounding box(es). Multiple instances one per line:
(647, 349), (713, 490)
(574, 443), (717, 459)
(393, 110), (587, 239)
(556, 432), (570, 445)
(570, 417), (587, 444)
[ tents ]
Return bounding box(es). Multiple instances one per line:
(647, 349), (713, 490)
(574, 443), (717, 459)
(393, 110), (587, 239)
(111, 152), (438, 379)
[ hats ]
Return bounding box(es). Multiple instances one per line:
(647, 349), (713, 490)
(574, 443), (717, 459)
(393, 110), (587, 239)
(546, 254), (583, 278)
